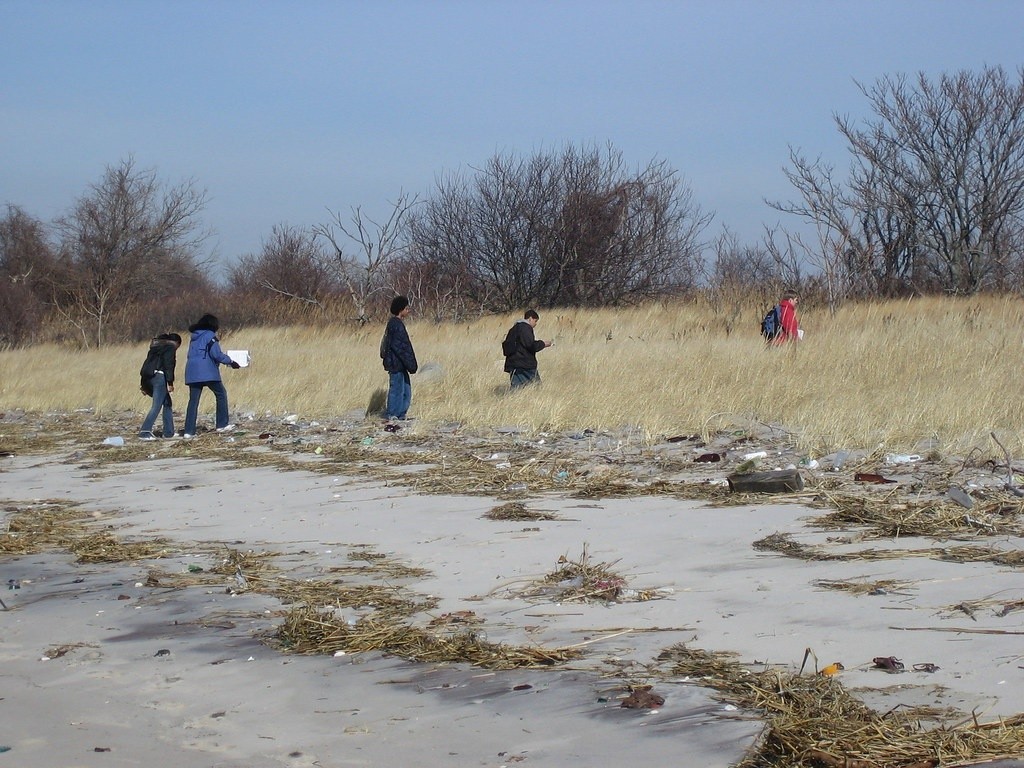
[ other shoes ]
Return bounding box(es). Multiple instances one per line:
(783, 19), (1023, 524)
(139, 433), (156, 441)
(162, 433), (180, 439)
(216, 424), (236, 433)
(183, 434), (197, 438)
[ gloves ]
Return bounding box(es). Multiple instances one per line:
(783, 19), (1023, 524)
(232, 361), (239, 369)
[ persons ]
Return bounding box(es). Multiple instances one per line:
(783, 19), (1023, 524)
(379, 295), (418, 423)
(183, 313), (241, 439)
(136, 332), (182, 441)
(504, 309), (551, 391)
(766, 290), (799, 347)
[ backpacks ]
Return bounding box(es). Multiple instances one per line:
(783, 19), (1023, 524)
(761, 307), (782, 341)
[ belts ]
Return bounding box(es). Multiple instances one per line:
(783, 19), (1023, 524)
(154, 369), (164, 374)
